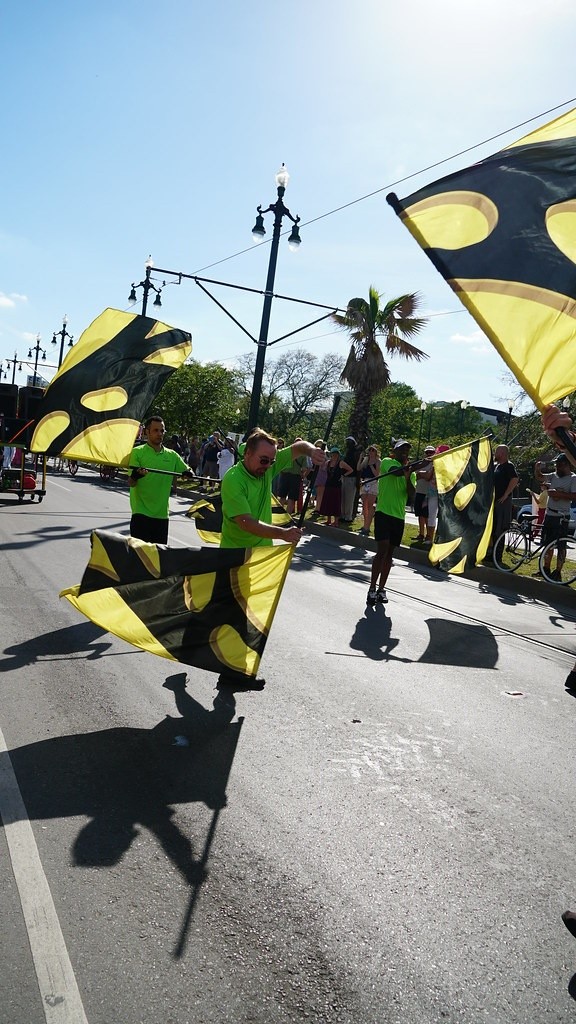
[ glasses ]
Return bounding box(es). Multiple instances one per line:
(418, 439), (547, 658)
(368, 449), (376, 452)
(251, 450), (276, 466)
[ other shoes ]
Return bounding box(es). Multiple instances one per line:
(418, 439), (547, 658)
(210, 488), (216, 493)
(201, 488), (207, 493)
(532, 567), (551, 577)
(215, 671), (266, 688)
(544, 569), (561, 582)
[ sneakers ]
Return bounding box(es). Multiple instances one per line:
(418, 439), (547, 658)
(366, 588), (380, 605)
(376, 590), (388, 602)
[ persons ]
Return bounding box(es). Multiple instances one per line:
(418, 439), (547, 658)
(408, 445), (450, 543)
(483, 444), (519, 562)
(0, 445), (22, 468)
(542, 404), (576, 467)
(216, 430), (325, 691)
(171, 431), (254, 495)
(272, 436), (380, 535)
(530, 483), (549, 539)
(532, 453), (576, 579)
(367, 441), (433, 603)
(127, 415), (196, 544)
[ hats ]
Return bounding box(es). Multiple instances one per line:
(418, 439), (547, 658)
(365, 444), (382, 460)
(436, 446), (449, 453)
(423, 446), (435, 452)
(345, 436), (357, 448)
(393, 441), (412, 452)
(550, 452), (570, 463)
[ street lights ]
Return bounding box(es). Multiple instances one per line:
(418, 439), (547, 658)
(458, 399), (469, 446)
(127, 253), (163, 317)
(562, 395), (571, 414)
(50, 311), (75, 372)
(5, 349), (24, 385)
(26, 331), (46, 386)
(245, 161), (304, 441)
(0, 361), (9, 386)
(415, 401), (427, 461)
(503, 397), (516, 444)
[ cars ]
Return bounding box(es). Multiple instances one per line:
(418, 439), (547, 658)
(515, 500), (576, 538)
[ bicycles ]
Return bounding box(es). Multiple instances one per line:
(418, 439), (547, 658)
(492, 505), (576, 584)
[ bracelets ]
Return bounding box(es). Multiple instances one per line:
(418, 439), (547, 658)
(343, 474), (345, 477)
(556, 431), (575, 450)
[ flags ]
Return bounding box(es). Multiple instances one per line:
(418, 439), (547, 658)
(185, 487), (293, 545)
(58, 528), (292, 680)
(27, 307), (192, 466)
(428, 439), (495, 573)
(400, 102), (576, 407)
